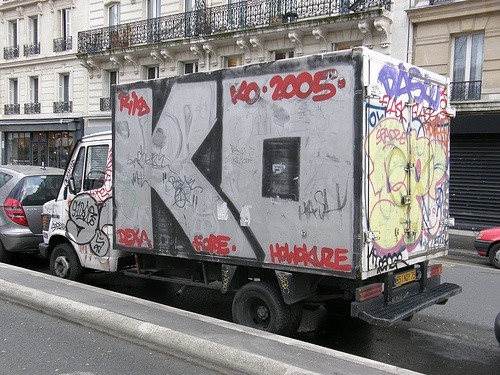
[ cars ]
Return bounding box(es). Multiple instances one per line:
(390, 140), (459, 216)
(0, 165), (67, 265)
(475, 227), (500, 265)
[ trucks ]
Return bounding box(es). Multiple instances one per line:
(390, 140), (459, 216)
(40, 46), (462, 333)
